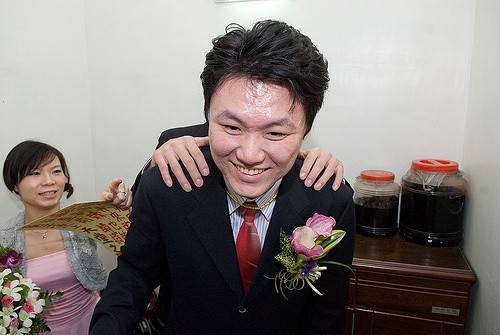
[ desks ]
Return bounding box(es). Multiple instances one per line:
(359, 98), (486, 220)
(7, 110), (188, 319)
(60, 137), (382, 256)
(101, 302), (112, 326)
(349, 237), (478, 333)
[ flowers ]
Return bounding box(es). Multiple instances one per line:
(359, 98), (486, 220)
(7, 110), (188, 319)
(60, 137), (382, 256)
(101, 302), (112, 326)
(0, 243), (65, 335)
(271, 211), (359, 300)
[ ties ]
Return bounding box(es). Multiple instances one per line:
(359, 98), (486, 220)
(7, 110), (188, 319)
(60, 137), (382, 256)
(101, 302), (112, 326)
(234, 203), (263, 296)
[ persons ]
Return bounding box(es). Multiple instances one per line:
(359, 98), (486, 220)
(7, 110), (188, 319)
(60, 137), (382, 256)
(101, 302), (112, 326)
(130, 120), (344, 195)
(0, 140), (134, 335)
(88, 19), (356, 335)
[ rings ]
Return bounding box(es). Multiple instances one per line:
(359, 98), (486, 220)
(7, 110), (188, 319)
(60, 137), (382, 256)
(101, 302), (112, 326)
(116, 190), (127, 196)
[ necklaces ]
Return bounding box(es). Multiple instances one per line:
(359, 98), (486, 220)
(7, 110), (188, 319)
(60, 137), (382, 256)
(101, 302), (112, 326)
(226, 190), (279, 210)
(32, 230), (49, 239)
